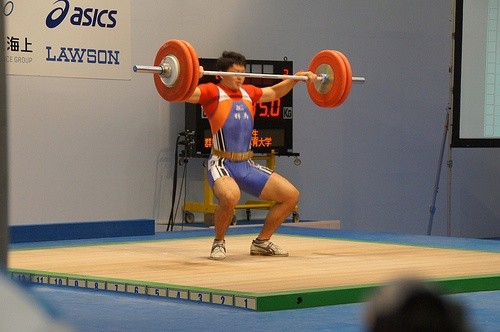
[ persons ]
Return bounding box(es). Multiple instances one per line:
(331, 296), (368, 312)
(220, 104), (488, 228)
(185, 50), (317, 260)
(363, 278), (471, 332)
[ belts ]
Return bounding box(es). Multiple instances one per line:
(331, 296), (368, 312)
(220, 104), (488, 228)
(210, 149), (253, 162)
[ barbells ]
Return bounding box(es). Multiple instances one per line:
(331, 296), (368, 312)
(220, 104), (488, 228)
(133, 38), (366, 109)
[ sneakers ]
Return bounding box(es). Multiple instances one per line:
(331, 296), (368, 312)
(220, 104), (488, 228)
(250, 239), (289, 257)
(210, 240), (226, 259)
